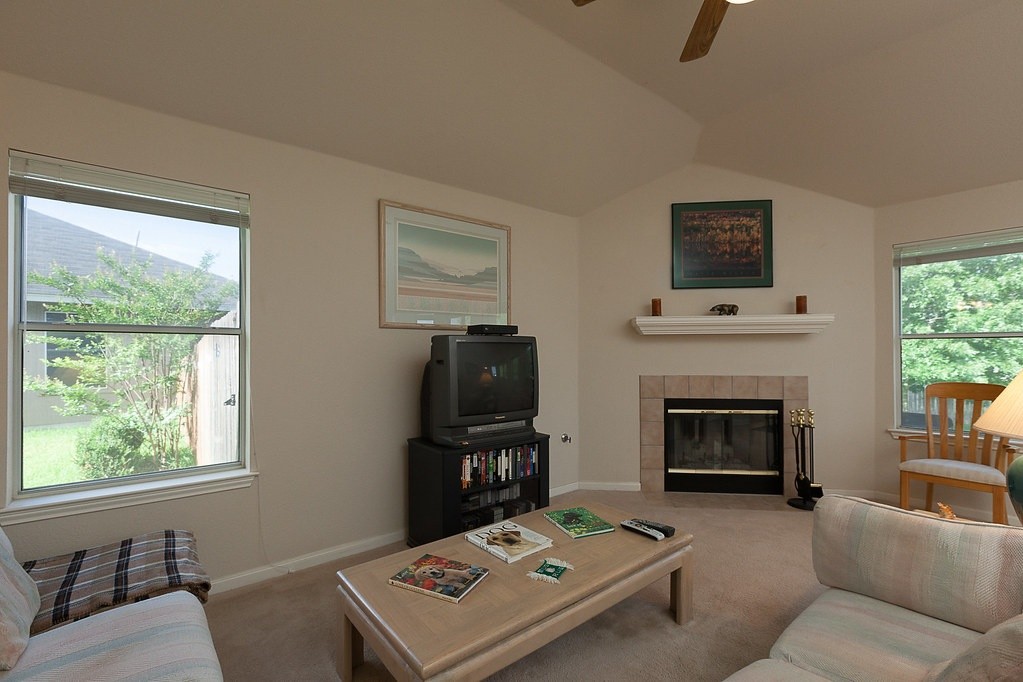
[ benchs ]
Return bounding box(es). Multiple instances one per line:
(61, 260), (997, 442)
(16, 528), (211, 635)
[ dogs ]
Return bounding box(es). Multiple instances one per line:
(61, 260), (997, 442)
(414, 565), (478, 589)
(562, 513), (583, 525)
(486, 531), (541, 557)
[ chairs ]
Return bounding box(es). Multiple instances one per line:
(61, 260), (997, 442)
(897, 382), (1011, 525)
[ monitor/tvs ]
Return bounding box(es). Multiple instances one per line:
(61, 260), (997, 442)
(421, 335), (539, 448)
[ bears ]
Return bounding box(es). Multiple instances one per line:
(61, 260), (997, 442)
(710, 304), (739, 316)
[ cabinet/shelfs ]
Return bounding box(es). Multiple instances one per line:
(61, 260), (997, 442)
(405, 432), (551, 549)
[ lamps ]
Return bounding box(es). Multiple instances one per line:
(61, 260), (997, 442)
(971, 369), (1023, 527)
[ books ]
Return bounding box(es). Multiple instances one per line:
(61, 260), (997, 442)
(459, 443), (540, 533)
(465, 519), (554, 565)
(543, 506), (616, 540)
(386, 553), (490, 604)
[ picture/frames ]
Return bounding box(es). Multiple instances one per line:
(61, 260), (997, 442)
(671, 198), (774, 288)
(377, 198), (513, 332)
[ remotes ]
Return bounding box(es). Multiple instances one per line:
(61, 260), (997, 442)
(620, 518), (675, 541)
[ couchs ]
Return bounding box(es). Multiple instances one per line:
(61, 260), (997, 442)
(720, 493), (1023, 682)
(0, 523), (224, 682)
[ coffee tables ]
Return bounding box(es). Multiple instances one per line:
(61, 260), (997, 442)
(333, 499), (694, 682)
